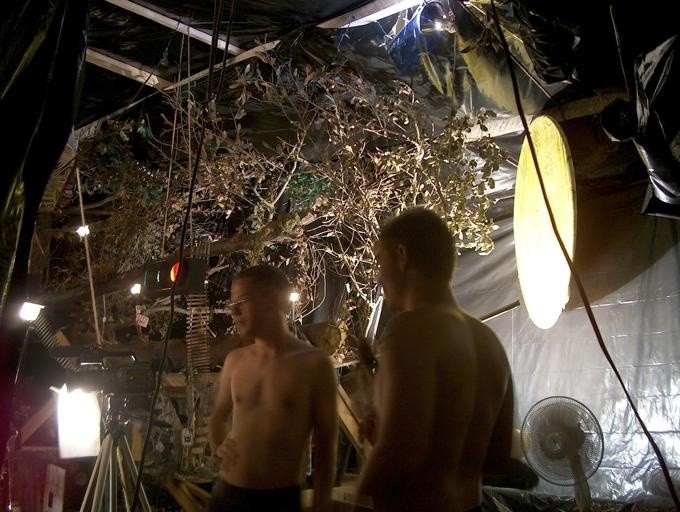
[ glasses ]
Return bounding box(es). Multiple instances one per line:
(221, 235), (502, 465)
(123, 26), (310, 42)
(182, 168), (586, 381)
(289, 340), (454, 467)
(225, 298), (248, 314)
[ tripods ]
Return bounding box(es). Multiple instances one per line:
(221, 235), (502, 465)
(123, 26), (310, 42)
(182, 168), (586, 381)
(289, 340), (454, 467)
(80, 429), (153, 512)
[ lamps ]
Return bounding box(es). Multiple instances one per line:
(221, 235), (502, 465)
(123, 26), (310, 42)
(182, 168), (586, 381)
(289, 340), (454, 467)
(418, 0), (459, 35)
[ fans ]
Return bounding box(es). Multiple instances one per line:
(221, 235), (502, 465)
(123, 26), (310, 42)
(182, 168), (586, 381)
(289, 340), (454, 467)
(520, 395), (604, 512)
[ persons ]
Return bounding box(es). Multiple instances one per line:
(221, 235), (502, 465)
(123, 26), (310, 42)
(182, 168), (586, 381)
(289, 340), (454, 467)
(348, 205), (516, 511)
(200, 263), (340, 511)
